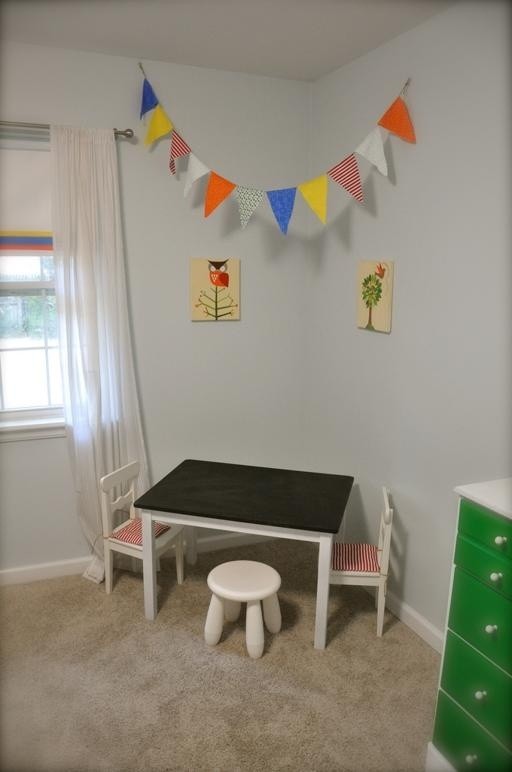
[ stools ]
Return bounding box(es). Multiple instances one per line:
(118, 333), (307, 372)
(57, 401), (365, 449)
(202, 558), (284, 661)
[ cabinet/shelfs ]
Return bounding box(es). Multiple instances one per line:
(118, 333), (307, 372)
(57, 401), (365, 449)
(421, 473), (512, 772)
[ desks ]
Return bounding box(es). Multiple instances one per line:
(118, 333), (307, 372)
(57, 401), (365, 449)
(130, 457), (355, 653)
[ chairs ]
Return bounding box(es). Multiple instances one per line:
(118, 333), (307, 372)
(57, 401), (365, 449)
(95, 458), (187, 621)
(328, 485), (397, 640)
